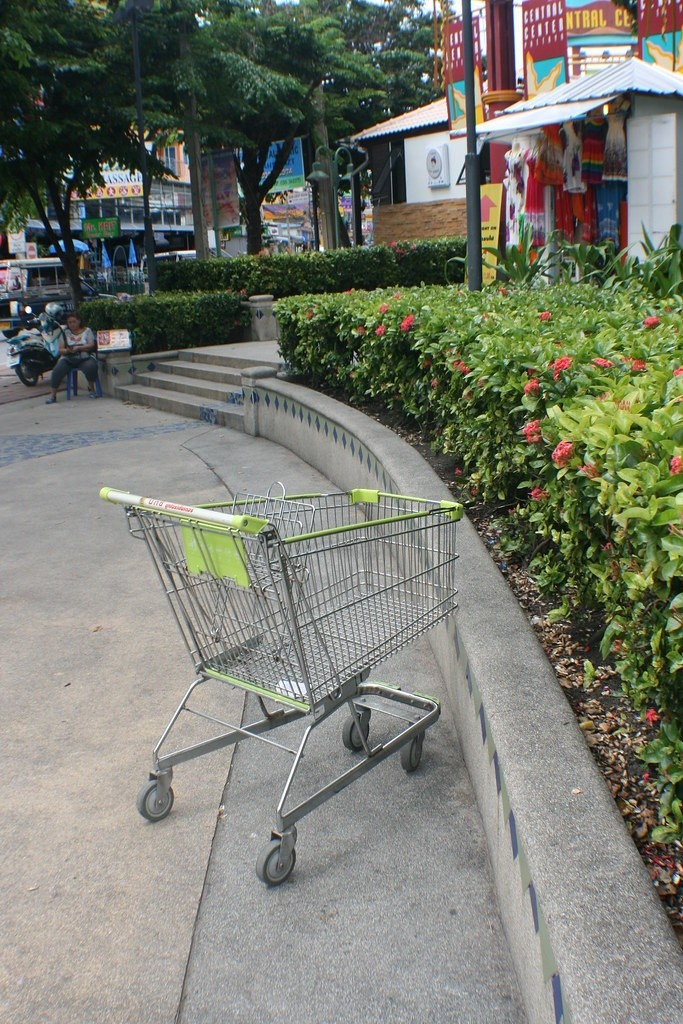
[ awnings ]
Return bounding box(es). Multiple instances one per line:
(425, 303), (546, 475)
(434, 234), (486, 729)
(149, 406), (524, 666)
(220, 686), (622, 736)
(450, 95), (632, 141)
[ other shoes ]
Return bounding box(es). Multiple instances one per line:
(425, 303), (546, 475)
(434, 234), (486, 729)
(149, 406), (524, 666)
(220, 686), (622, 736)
(87, 389), (96, 398)
(45, 397), (57, 404)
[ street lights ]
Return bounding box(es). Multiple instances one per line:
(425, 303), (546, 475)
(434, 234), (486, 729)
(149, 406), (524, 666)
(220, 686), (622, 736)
(306, 145), (355, 249)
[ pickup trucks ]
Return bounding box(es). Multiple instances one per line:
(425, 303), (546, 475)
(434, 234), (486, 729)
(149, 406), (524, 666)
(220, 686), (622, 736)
(0, 276), (118, 339)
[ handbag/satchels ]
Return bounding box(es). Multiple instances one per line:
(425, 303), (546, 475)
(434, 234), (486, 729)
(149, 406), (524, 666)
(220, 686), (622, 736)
(67, 344), (89, 360)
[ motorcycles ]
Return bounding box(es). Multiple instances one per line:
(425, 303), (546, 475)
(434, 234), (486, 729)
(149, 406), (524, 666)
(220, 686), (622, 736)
(1, 301), (68, 387)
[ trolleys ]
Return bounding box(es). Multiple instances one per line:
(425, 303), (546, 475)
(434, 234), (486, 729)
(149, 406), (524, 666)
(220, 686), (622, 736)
(100, 482), (465, 888)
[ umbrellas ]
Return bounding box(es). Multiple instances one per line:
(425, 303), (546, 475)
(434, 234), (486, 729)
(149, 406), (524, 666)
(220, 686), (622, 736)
(50, 239), (88, 253)
(102, 245), (111, 288)
(129, 240), (137, 268)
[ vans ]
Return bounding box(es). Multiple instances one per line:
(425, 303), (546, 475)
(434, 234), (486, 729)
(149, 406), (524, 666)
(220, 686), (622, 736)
(0, 257), (85, 299)
(140, 247), (233, 297)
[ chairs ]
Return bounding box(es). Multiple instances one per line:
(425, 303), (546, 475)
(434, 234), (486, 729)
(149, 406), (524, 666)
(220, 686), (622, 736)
(67, 336), (102, 400)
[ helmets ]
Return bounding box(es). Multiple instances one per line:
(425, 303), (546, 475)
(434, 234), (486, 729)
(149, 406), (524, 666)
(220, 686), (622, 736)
(45, 302), (63, 317)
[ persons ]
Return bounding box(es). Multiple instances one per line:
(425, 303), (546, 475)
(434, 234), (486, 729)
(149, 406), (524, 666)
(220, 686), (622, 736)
(46, 314), (96, 403)
